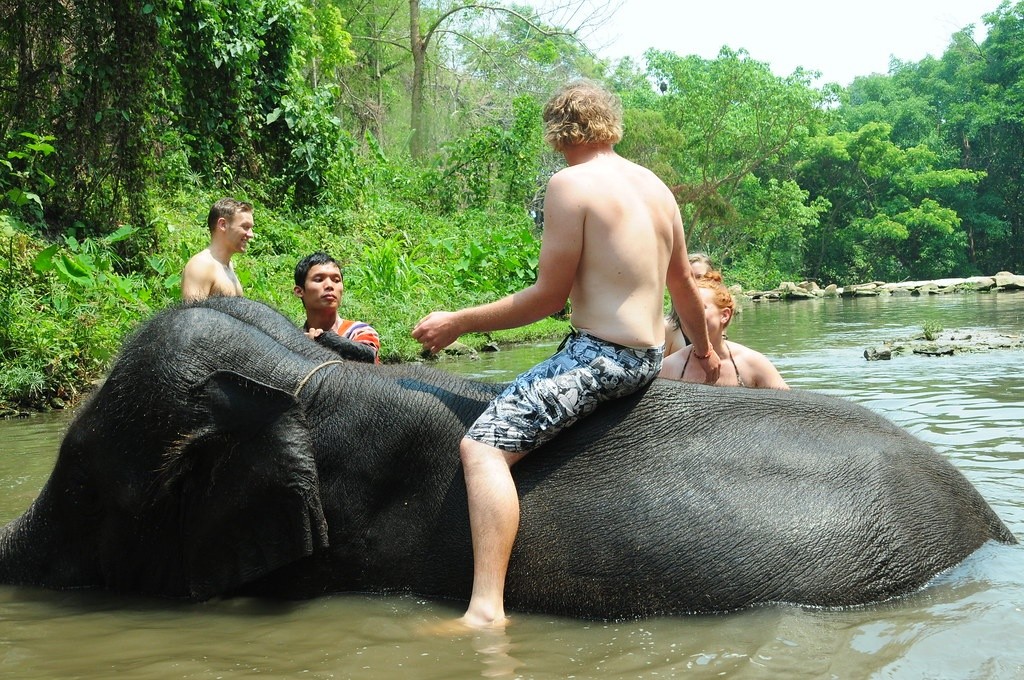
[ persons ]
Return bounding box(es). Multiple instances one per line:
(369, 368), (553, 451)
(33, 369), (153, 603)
(181, 197), (256, 302)
(655, 251), (791, 389)
(293, 251), (380, 364)
(411, 76), (721, 629)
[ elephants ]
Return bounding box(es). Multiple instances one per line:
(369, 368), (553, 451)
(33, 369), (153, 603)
(1, 293), (1023, 628)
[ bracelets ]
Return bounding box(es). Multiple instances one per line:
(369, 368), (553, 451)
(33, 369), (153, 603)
(693, 342), (713, 359)
